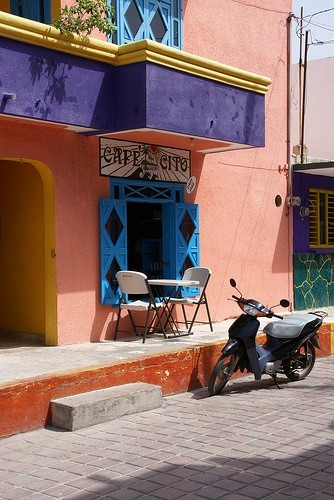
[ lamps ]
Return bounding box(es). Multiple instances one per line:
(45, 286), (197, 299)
(286, 195), (301, 208)
(300, 205), (310, 218)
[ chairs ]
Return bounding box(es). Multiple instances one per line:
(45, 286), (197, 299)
(163, 266), (213, 333)
(113, 270), (167, 343)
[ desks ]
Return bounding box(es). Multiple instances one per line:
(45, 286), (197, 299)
(141, 279), (200, 339)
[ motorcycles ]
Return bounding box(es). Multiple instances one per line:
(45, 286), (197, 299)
(208, 278), (328, 398)
